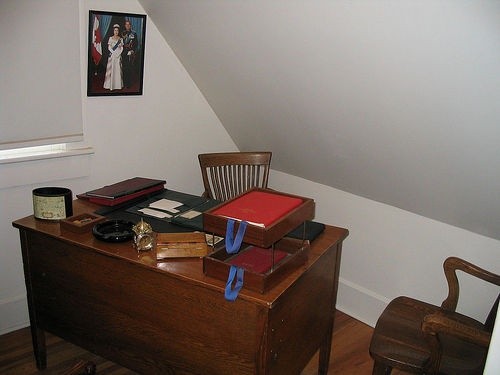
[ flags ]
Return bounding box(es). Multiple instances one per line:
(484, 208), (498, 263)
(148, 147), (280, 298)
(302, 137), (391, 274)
(91, 16), (103, 65)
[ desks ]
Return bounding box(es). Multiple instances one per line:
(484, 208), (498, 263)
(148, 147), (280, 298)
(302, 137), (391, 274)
(12, 189), (349, 375)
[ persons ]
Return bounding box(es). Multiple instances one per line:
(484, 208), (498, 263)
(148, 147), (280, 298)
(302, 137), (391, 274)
(103, 21), (138, 91)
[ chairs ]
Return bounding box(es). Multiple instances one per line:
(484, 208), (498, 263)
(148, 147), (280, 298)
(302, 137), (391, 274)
(369, 256), (500, 375)
(197, 152), (272, 202)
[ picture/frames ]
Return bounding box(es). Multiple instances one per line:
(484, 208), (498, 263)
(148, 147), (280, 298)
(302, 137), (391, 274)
(86, 9), (147, 97)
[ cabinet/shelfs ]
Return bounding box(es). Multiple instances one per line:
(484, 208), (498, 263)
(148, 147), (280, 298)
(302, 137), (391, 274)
(201, 187), (315, 295)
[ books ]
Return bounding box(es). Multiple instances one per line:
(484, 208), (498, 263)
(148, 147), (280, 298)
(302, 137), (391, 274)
(225, 245), (288, 274)
(213, 189), (304, 228)
(75, 176), (166, 206)
